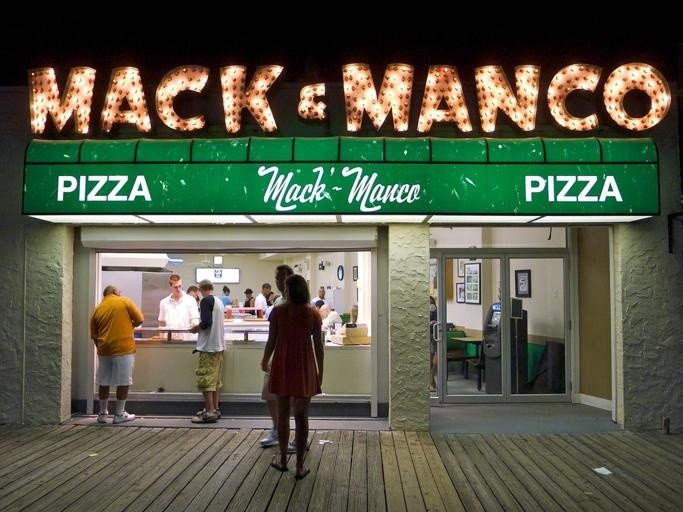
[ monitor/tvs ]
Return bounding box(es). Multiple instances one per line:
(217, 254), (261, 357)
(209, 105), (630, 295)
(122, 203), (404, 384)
(489, 311), (501, 324)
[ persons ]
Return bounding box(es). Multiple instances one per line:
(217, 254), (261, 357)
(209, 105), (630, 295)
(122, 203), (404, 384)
(259, 274), (324, 480)
(183, 281), (344, 338)
(428, 296), (438, 391)
(155, 273), (200, 340)
(88, 284), (144, 424)
(256, 264), (311, 453)
(185, 277), (227, 423)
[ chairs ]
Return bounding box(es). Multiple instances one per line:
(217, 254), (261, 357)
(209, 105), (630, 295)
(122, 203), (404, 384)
(432, 328), (563, 394)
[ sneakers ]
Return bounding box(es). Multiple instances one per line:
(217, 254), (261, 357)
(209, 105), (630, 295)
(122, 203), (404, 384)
(191, 408), (221, 423)
(112, 410), (136, 424)
(288, 438), (309, 454)
(97, 410), (108, 423)
(260, 427), (279, 447)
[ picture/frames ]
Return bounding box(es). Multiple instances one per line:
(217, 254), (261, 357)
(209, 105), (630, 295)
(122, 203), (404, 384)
(352, 266), (357, 281)
(337, 265), (343, 280)
(454, 254), (531, 304)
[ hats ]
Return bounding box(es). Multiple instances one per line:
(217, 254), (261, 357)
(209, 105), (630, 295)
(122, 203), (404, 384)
(199, 279), (212, 287)
